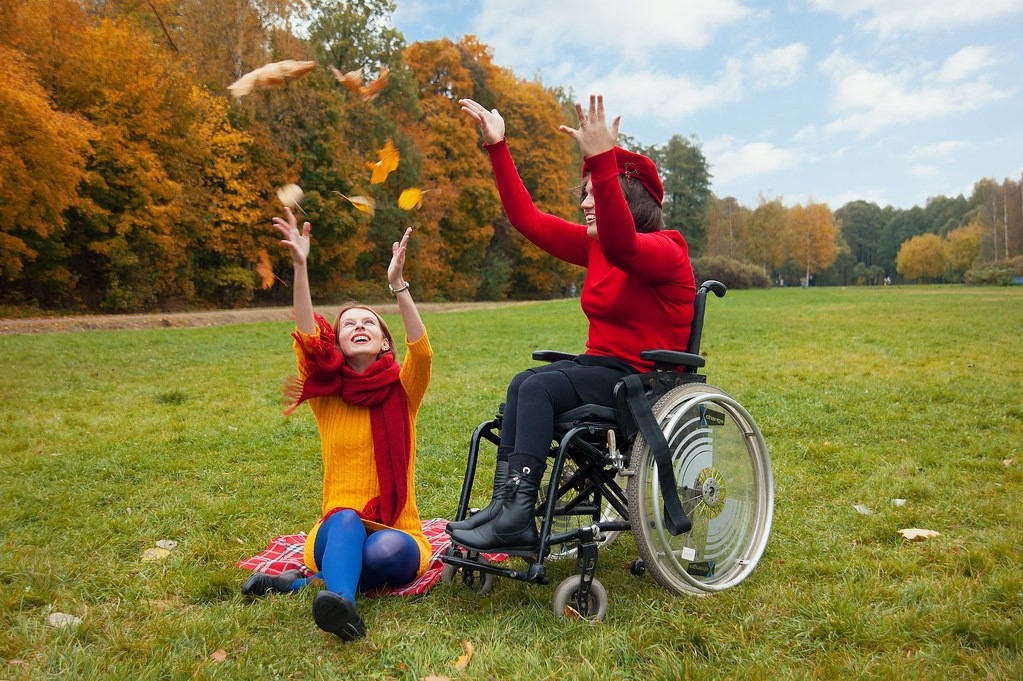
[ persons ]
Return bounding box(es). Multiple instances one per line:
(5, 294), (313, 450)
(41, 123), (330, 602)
(447, 94), (695, 556)
(241, 206), (432, 642)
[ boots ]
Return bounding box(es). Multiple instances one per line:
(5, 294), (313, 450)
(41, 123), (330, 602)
(445, 454), (511, 534)
(451, 452), (546, 556)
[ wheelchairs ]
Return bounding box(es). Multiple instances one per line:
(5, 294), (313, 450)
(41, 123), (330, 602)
(440, 280), (774, 624)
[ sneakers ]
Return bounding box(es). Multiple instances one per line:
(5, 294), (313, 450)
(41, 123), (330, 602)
(242, 569), (300, 597)
(312, 589), (365, 641)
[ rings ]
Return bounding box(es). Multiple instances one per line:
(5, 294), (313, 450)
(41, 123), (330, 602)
(580, 121), (587, 126)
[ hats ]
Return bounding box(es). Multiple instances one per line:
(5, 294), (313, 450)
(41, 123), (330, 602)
(579, 146), (664, 208)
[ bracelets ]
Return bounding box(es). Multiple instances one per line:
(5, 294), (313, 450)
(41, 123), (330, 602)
(389, 281), (410, 296)
